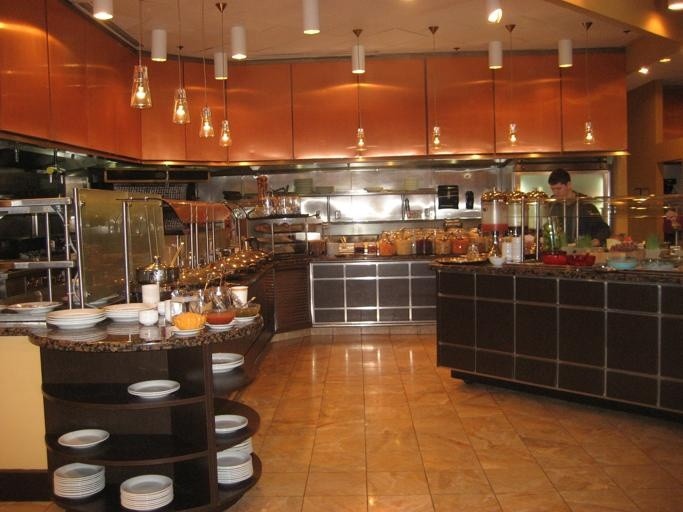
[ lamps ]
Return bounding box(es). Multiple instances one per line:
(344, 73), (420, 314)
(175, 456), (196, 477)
(152, 29), (168, 62)
(174, 2), (190, 127)
(199, 1), (215, 140)
(352, 28), (365, 74)
(428, 25), (441, 150)
(219, 3), (231, 145)
(214, 52), (229, 80)
(93, 2), (115, 21)
(505, 24), (519, 148)
(488, 41), (503, 70)
(559, 38), (573, 68)
(356, 74), (366, 150)
(232, 25), (246, 60)
(354, 151), (366, 162)
(303, 1), (321, 36)
(130, 1), (152, 109)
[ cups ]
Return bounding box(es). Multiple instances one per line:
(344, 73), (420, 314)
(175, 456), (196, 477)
(141, 284), (160, 303)
(501, 235), (520, 262)
(159, 285), (248, 321)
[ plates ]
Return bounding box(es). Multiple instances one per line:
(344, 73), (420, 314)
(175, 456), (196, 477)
(169, 313), (259, 336)
(293, 178), (334, 195)
(325, 242), (338, 256)
(215, 438), (254, 486)
(47, 302), (158, 328)
(5, 300), (62, 313)
(53, 462), (105, 499)
(57, 428), (109, 448)
(210, 350), (245, 373)
(213, 414), (248, 434)
(126, 379), (180, 398)
(363, 175), (433, 193)
(118, 473), (173, 512)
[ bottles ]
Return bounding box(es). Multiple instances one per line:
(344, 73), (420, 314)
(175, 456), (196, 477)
(367, 242), (377, 256)
(622, 237), (633, 251)
(254, 193), (300, 215)
(353, 242), (364, 255)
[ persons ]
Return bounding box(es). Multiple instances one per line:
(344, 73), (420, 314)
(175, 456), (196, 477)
(547, 169), (610, 248)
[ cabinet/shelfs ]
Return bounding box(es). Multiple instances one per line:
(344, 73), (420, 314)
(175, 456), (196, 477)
(290, 189), (482, 225)
(1, 0), (87, 154)
(224, 198), (309, 258)
(0, 192), (166, 323)
(561, 48), (630, 160)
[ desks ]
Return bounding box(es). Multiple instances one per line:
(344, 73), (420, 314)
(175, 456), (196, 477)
(25, 310), (264, 511)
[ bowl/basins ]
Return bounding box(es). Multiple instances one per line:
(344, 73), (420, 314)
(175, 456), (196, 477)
(606, 256), (638, 269)
(173, 302), (264, 330)
(542, 254), (567, 265)
(334, 254), (346, 257)
(134, 256), (178, 286)
(566, 255), (595, 266)
(488, 257), (505, 266)
